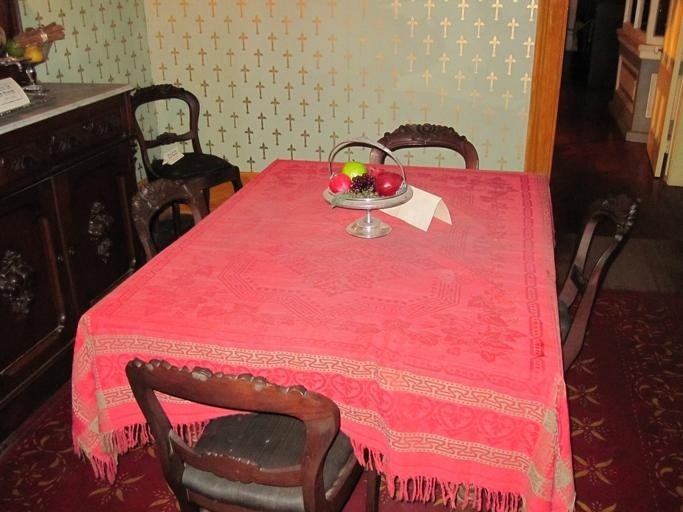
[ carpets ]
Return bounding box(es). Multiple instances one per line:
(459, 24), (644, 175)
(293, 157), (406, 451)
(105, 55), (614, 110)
(0, 260), (683, 509)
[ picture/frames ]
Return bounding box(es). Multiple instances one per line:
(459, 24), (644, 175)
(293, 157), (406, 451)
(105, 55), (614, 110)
(0, 0), (37, 90)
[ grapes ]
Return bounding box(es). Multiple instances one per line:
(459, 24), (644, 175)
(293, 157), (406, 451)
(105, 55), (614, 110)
(350, 172), (375, 195)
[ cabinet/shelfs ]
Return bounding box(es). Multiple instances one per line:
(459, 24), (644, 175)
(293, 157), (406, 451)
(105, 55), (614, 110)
(0, 78), (140, 444)
(608, 1), (671, 147)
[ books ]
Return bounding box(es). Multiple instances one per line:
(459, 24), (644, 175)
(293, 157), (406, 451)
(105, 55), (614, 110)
(0, 77), (30, 118)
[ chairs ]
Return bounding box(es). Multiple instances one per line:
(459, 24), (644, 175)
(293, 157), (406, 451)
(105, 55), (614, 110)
(116, 357), (386, 511)
(130, 175), (207, 270)
(551, 181), (643, 381)
(127, 76), (241, 215)
(368, 122), (482, 172)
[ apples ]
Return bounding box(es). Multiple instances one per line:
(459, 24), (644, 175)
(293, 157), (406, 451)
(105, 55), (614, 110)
(342, 162), (367, 179)
(375, 172), (402, 197)
(328, 174), (352, 193)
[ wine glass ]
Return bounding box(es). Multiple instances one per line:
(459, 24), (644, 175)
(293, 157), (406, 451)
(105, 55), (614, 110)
(323, 185), (413, 239)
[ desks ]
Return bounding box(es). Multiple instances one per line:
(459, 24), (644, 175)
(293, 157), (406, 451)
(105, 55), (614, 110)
(63, 153), (581, 510)
